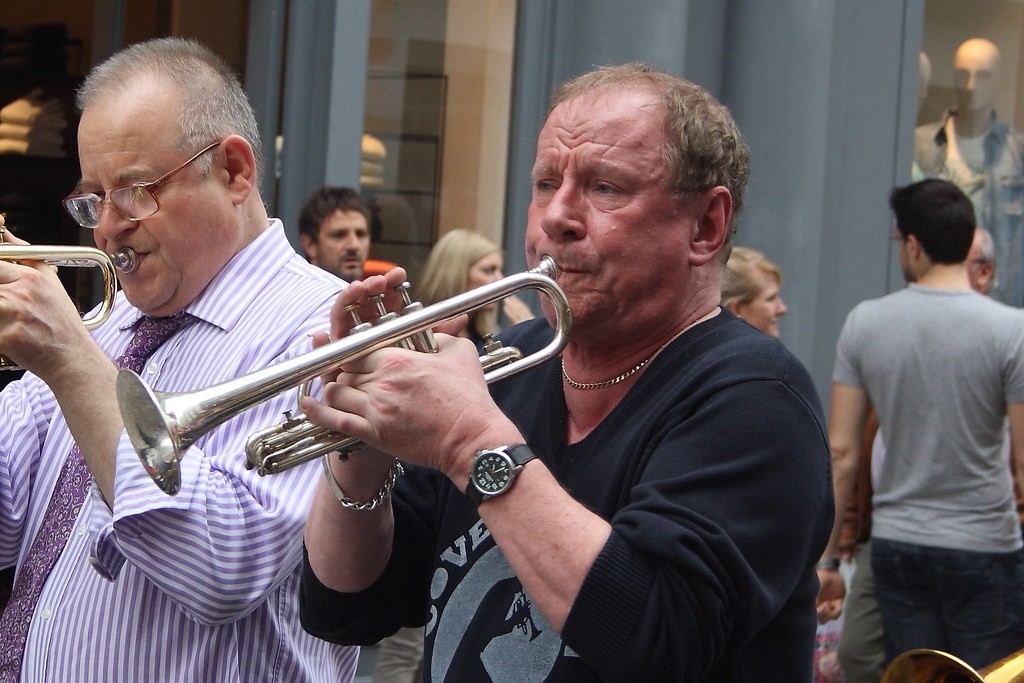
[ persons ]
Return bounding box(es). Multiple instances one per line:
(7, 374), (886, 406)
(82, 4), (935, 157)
(301, 60), (836, 683)
(0, 38), (370, 683)
(908, 36), (1024, 308)
(296, 186), (376, 281)
(831, 224), (997, 683)
(814, 175), (1022, 670)
(415, 227), (538, 342)
(716, 242), (787, 339)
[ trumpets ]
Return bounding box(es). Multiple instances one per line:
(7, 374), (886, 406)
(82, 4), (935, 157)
(0, 225), (140, 371)
(879, 648), (1024, 682)
(117, 255), (574, 498)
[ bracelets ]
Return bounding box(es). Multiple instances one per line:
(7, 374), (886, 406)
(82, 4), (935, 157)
(816, 556), (841, 572)
(320, 453), (404, 515)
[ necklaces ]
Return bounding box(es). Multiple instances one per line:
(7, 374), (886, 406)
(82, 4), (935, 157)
(559, 355), (653, 392)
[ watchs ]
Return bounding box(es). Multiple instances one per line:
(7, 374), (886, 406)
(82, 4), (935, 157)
(465, 442), (539, 508)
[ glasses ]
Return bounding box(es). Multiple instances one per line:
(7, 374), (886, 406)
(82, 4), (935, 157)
(59, 142), (221, 228)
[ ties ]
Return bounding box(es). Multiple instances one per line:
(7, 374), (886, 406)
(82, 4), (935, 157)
(0, 316), (200, 683)
